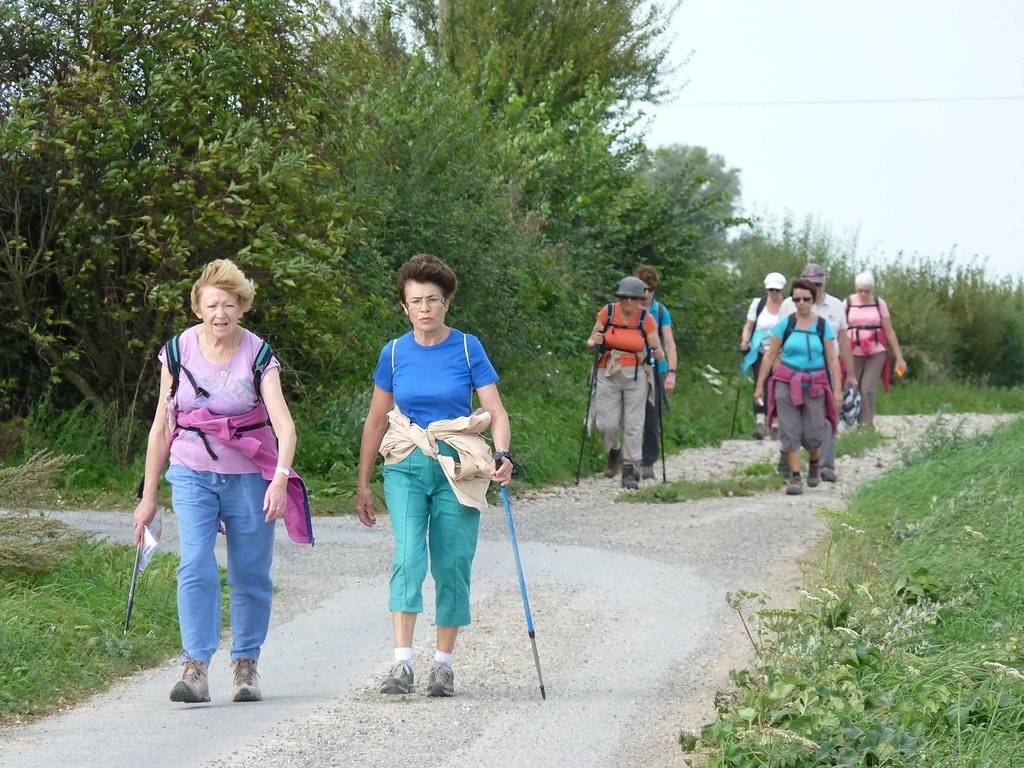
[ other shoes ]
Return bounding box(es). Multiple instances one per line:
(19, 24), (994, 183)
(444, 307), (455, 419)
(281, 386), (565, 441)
(807, 458), (820, 487)
(778, 453), (790, 478)
(786, 476), (804, 494)
(752, 424), (764, 440)
(770, 426), (779, 440)
(821, 471), (836, 482)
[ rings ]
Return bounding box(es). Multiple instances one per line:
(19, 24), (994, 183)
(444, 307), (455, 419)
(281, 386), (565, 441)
(277, 507), (280, 510)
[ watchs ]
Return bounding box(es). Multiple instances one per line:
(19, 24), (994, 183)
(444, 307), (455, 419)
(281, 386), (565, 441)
(668, 369), (677, 373)
(503, 450), (514, 464)
(276, 467), (290, 476)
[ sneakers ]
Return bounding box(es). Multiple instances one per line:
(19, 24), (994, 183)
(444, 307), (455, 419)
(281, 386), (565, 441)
(642, 465), (654, 479)
(170, 650), (211, 702)
(380, 660), (415, 693)
(229, 658), (266, 701)
(426, 661), (455, 697)
(621, 465), (637, 490)
(603, 446), (621, 478)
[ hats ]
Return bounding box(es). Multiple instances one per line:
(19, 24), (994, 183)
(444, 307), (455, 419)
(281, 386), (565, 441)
(802, 264), (826, 283)
(764, 272), (786, 289)
(614, 277), (648, 297)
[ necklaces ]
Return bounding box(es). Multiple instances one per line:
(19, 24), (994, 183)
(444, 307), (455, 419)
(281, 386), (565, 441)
(204, 328), (237, 376)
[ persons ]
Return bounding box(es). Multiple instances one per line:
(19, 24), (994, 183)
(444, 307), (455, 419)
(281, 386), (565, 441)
(754, 281), (842, 496)
(741, 272), (787, 442)
(635, 266), (678, 479)
(355, 254), (514, 697)
(586, 277), (665, 489)
(133, 259), (296, 701)
(840, 270), (907, 425)
(754, 263), (859, 482)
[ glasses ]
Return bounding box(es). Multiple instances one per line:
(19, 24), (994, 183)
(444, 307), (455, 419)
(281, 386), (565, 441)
(792, 297), (813, 302)
(856, 288), (872, 294)
(767, 288), (780, 292)
(815, 283), (822, 287)
(644, 287), (654, 293)
(621, 296), (638, 300)
(405, 294), (446, 308)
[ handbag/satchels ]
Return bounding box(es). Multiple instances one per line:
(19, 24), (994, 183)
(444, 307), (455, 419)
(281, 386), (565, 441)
(840, 387), (862, 427)
